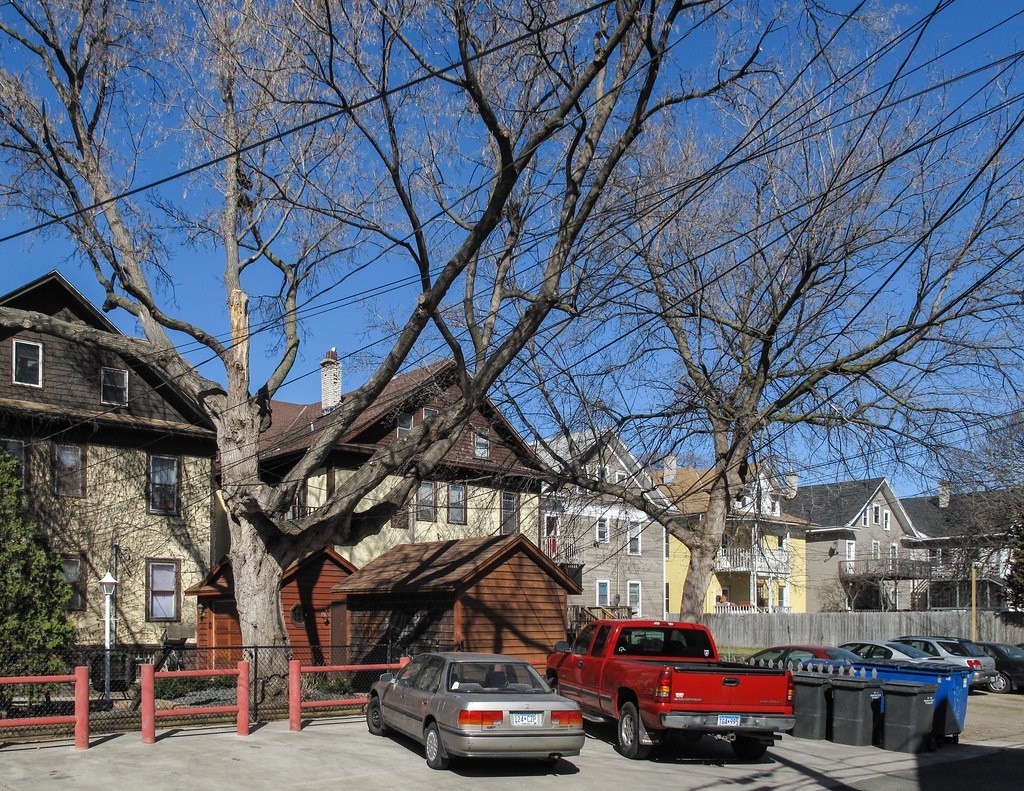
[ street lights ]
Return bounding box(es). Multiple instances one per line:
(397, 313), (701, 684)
(98, 568), (120, 702)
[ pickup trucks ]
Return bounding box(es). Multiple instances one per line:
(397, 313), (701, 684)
(543, 616), (798, 761)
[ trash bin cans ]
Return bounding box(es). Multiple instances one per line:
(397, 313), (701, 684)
(793, 673), (833, 740)
(830, 676), (883, 747)
(802, 659), (974, 744)
(882, 683), (938, 755)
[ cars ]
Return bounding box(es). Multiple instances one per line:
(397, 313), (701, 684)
(837, 639), (955, 665)
(744, 643), (869, 664)
(365, 651), (587, 772)
(888, 633), (1001, 686)
(949, 641), (1024, 694)
